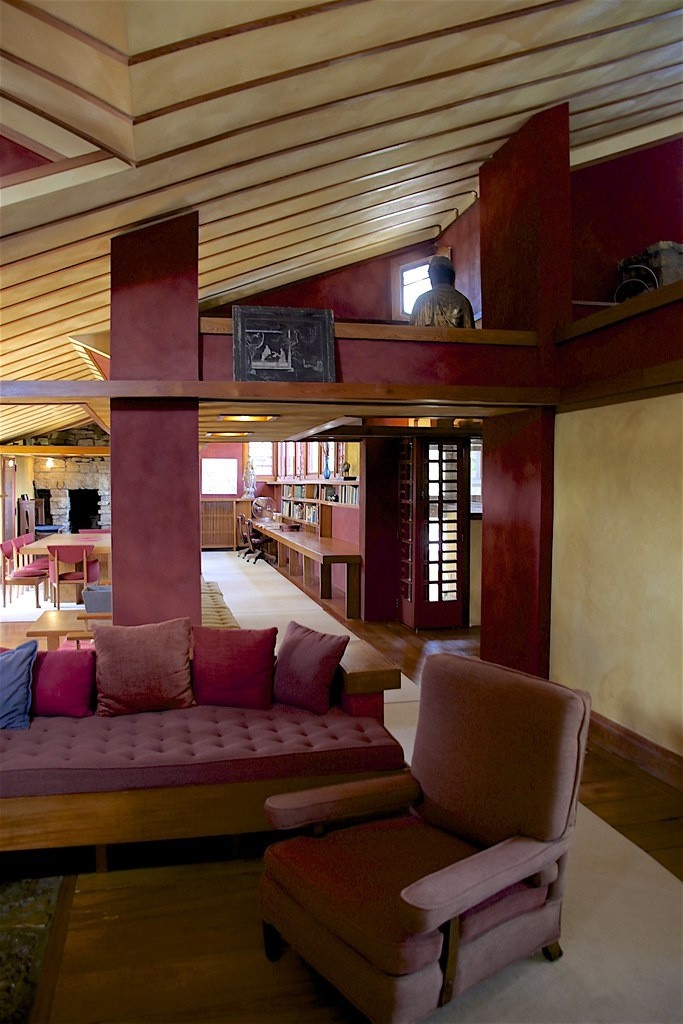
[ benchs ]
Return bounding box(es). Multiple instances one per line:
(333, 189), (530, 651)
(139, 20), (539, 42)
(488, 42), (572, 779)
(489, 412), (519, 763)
(202, 581), (241, 629)
(0, 641), (411, 872)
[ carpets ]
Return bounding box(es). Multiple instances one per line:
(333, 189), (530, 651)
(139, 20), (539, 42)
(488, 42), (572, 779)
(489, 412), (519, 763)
(0, 870), (78, 1024)
(0, 584), (85, 622)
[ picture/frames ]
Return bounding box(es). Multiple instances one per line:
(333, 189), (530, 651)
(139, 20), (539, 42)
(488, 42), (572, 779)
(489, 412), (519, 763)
(230, 305), (336, 384)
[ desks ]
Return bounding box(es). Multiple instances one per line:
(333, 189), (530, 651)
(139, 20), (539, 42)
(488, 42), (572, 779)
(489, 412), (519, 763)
(19, 533), (111, 603)
(25, 610), (112, 651)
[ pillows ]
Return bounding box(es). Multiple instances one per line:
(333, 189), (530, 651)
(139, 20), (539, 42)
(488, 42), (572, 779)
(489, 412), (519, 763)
(191, 624), (278, 710)
(90, 616), (197, 716)
(273, 621), (351, 715)
(0, 647), (95, 718)
(0, 638), (39, 730)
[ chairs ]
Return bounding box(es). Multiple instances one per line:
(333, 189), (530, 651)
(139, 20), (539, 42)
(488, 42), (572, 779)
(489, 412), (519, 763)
(262, 652), (590, 1024)
(0, 528), (111, 611)
(236, 514), (276, 565)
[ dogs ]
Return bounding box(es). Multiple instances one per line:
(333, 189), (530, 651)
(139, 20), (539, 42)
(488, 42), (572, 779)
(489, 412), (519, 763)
(339, 457), (350, 476)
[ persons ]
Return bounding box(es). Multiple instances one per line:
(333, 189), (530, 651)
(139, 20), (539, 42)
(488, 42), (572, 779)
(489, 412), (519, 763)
(408, 256), (475, 329)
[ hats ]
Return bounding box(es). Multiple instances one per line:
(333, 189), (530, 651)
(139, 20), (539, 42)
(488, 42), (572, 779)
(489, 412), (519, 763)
(428, 256), (454, 272)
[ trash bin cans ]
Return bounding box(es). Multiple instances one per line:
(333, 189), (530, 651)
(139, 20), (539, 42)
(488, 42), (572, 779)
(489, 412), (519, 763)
(81, 584), (112, 614)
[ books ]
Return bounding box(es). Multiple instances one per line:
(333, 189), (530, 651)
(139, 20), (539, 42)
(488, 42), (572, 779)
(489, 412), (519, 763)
(283, 484), (359, 505)
(282, 501), (318, 524)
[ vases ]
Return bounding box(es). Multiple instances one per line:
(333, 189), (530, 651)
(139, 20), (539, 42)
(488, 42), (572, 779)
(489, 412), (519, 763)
(322, 456), (330, 479)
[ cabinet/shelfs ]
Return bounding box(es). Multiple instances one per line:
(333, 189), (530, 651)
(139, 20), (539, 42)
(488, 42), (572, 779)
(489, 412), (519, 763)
(249, 481), (361, 619)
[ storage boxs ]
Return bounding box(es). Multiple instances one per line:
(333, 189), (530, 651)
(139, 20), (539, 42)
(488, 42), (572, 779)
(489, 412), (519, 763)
(82, 586), (111, 612)
